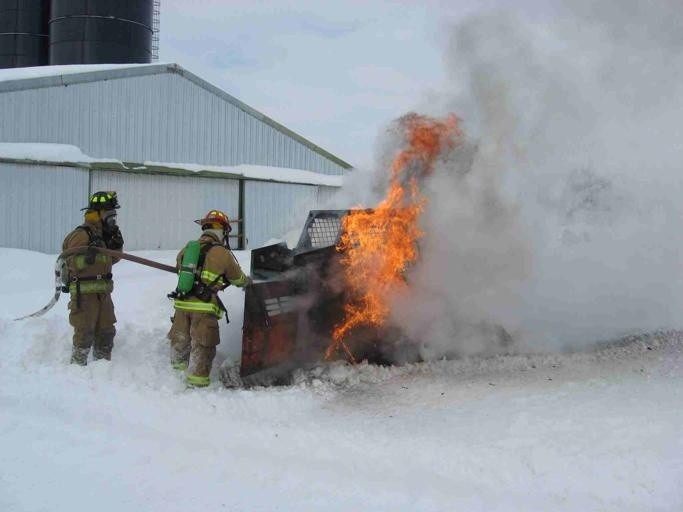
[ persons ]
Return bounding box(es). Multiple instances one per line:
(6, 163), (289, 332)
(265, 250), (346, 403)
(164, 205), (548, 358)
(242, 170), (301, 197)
(61, 191), (123, 365)
(168, 210), (252, 389)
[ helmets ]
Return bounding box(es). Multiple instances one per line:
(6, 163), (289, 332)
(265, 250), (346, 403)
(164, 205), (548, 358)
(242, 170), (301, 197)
(194, 209), (233, 232)
(87, 190), (120, 210)
